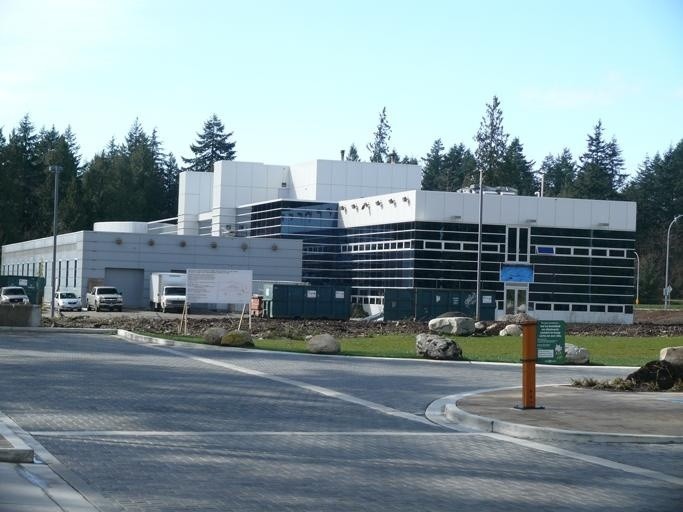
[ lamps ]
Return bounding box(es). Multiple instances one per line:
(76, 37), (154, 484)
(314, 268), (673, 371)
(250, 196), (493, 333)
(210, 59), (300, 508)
(278, 207), (332, 221)
(339, 197), (409, 211)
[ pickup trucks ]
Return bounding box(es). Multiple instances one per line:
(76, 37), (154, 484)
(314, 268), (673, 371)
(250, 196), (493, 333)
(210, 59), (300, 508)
(86, 286), (124, 313)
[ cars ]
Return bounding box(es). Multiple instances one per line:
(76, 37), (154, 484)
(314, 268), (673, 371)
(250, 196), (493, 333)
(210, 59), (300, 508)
(0, 286), (30, 306)
(54, 292), (82, 312)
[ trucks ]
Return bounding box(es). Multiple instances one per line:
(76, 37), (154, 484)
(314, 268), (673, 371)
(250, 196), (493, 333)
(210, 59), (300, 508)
(149, 271), (192, 315)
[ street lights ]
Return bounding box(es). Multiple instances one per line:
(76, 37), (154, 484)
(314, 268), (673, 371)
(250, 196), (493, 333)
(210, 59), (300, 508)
(472, 166), (484, 322)
(46, 163), (63, 318)
